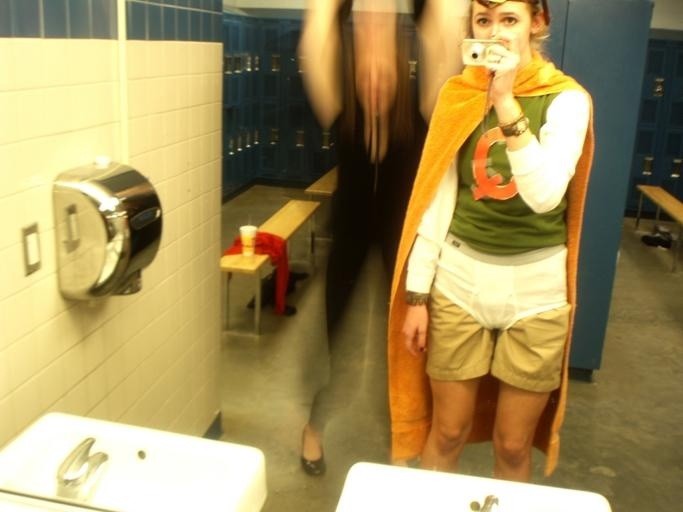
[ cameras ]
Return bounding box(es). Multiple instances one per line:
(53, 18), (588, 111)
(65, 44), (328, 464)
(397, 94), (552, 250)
(462, 39), (505, 69)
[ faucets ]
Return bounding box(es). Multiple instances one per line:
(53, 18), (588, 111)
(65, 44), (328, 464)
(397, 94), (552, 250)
(56, 438), (109, 498)
(470, 495), (498, 512)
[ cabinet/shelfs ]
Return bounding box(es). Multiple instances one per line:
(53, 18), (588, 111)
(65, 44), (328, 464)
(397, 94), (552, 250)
(220, 12), (333, 193)
(628, 30), (683, 222)
(0, 1), (226, 43)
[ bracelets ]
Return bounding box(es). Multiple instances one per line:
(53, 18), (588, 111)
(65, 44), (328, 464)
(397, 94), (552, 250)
(499, 113), (532, 138)
(403, 290), (432, 310)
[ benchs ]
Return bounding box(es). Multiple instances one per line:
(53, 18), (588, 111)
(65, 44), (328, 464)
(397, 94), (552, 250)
(303, 166), (339, 239)
(221, 199), (324, 337)
(637, 183), (682, 239)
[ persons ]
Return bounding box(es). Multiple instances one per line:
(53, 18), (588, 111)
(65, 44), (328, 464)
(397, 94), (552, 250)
(397, 1), (593, 485)
(294, 3), (471, 476)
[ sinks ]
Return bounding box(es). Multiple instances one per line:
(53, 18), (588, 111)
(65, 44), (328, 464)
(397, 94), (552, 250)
(335, 461), (610, 512)
(0, 413), (267, 512)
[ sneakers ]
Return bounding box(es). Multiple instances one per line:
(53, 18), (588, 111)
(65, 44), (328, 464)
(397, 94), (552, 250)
(642, 235), (671, 248)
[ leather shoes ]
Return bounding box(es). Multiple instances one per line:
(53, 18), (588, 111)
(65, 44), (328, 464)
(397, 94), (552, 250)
(300, 425), (326, 476)
